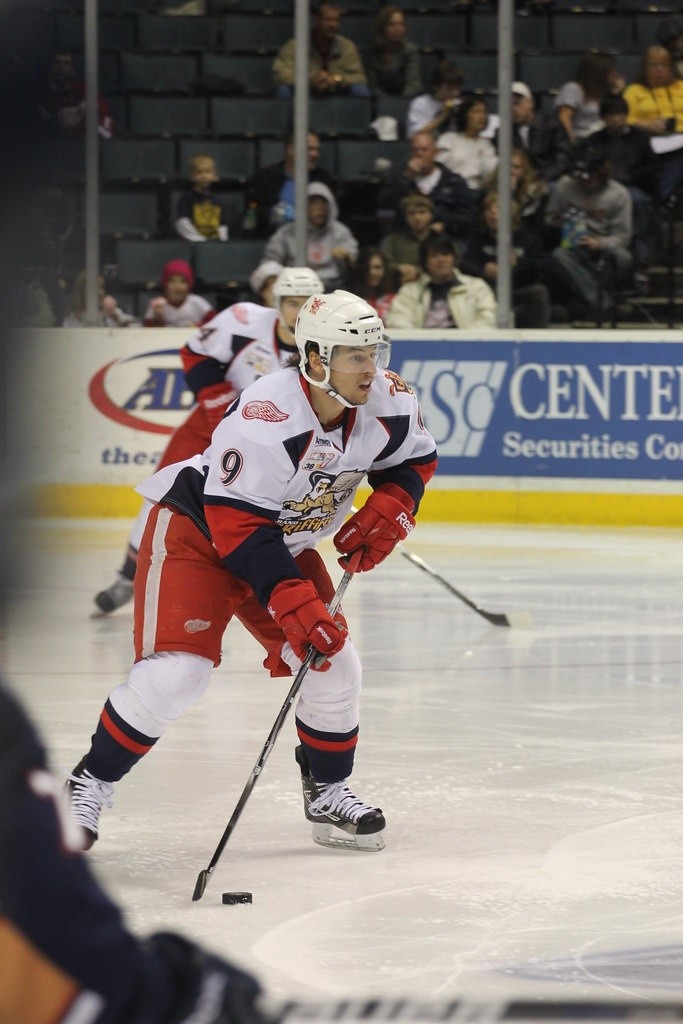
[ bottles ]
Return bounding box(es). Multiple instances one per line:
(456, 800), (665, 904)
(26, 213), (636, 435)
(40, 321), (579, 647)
(242, 202), (258, 235)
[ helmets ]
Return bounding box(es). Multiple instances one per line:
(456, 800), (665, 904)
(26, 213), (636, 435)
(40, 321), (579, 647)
(272, 266), (325, 337)
(294, 290), (392, 387)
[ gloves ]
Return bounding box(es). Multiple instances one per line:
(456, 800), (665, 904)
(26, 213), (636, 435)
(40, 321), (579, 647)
(200, 383), (242, 430)
(266, 578), (349, 672)
(334, 481), (415, 573)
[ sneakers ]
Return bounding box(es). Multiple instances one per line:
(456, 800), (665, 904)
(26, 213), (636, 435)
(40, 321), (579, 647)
(93, 570), (135, 613)
(300, 774), (386, 834)
(60, 754), (118, 852)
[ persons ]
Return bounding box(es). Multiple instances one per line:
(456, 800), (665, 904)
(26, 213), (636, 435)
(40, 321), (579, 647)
(176, 21), (683, 327)
(61, 289), (438, 852)
(273, 0), (369, 97)
(93, 267), (325, 613)
(0, 260), (218, 327)
(20, 48), (115, 138)
(0, 682), (275, 1024)
(360, 5), (431, 98)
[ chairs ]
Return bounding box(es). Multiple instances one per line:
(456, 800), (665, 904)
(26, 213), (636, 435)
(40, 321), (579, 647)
(20, 0), (683, 331)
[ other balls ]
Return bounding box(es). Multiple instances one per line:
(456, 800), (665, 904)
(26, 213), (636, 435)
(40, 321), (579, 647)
(222, 892), (252, 904)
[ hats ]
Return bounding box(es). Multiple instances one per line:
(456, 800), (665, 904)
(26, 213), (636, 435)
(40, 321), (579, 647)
(250, 259), (284, 294)
(510, 80), (532, 97)
(564, 140), (604, 194)
(186, 156), (218, 182)
(162, 260), (192, 288)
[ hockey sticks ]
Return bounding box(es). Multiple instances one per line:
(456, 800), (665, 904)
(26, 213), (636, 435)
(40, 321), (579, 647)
(350, 503), (509, 627)
(191, 546), (365, 901)
(255, 995), (683, 1024)
